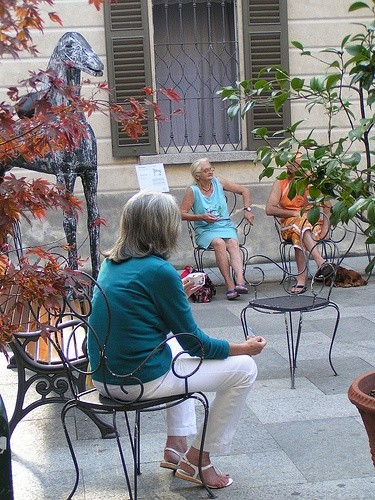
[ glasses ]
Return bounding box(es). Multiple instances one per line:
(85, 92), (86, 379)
(197, 167), (214, 174)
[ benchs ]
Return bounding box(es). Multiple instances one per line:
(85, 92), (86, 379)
(0, 259), (122, 439)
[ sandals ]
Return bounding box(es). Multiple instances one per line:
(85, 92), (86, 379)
(160, 447), (189, 470)
(175, 456), (232, 489)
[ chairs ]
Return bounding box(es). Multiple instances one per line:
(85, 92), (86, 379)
(185, 191), (252, 285)
(29, 270), (218, 499)
(240, 212), (357, 387)
(273, 215), (335, 284)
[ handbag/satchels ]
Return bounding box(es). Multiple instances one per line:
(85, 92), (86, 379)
(180, 266), (216, 303)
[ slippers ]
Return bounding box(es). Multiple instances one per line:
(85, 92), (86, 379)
(291, 284), (307, 294)
(235, 284), (248, 295)
(320, 261), (335, 275)
(226, 290), (239, 299)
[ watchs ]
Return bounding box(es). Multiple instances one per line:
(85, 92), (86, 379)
(244, 207), (252, 212)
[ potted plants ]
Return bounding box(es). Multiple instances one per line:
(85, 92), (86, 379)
(217, 1), (375, 463)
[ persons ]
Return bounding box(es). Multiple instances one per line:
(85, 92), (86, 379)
(87, 190), (267, 488)
(178, 158), (254, 301)
(266, 152), (334, 293)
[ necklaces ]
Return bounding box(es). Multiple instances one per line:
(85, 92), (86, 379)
(202, 185), (212, 192)
